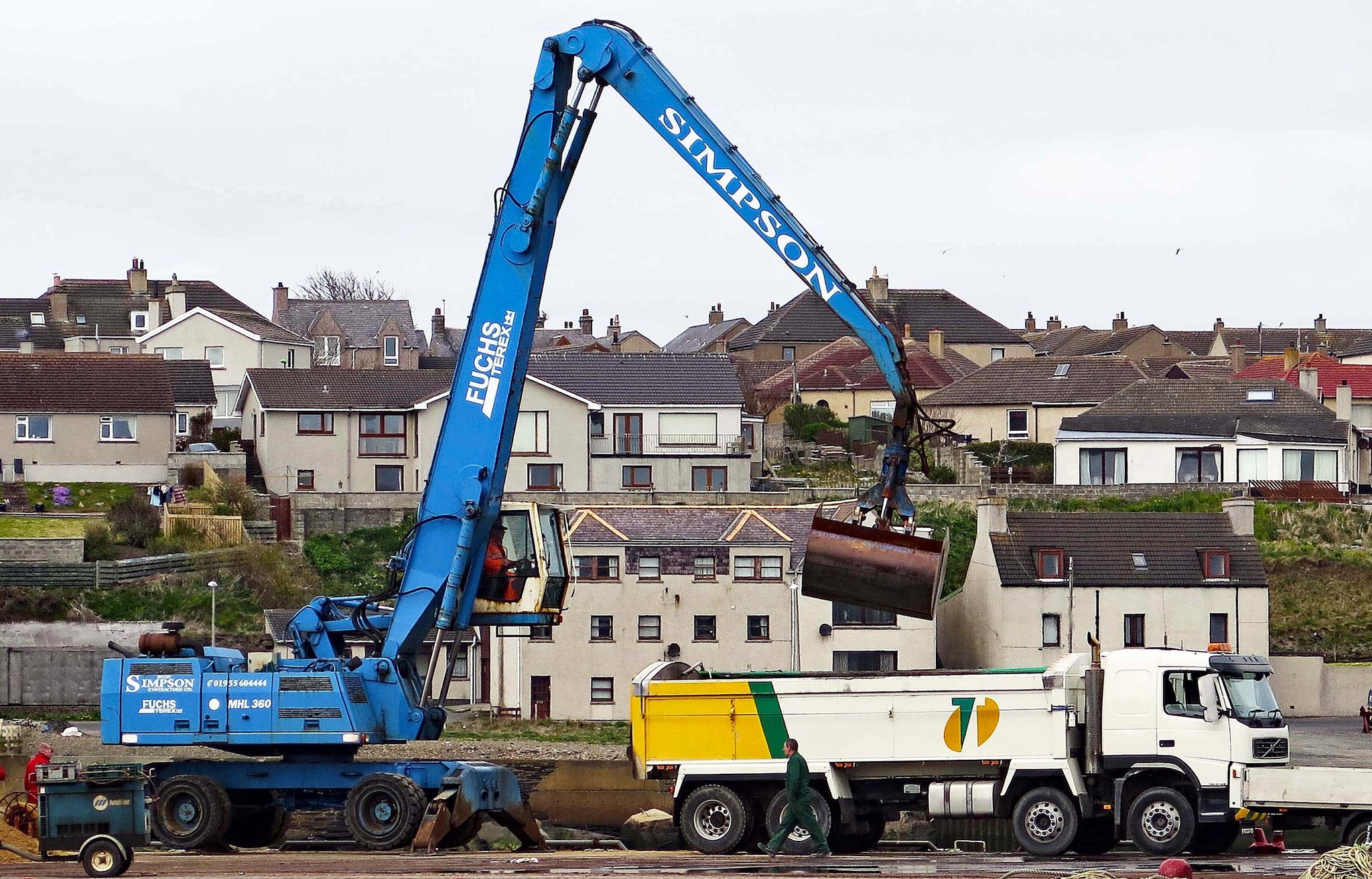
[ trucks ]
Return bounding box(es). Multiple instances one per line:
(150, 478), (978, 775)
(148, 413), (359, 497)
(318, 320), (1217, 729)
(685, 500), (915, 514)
(1226, 756), (1372, 854)
(623, 632), (1294, 861)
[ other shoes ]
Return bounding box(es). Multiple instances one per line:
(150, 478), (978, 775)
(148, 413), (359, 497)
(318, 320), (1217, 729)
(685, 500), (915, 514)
(758, 843), (775, 857)
(810, 852), (831, 858)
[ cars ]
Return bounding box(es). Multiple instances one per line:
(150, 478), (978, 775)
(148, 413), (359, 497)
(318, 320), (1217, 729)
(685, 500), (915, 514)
(184, 442), (220, 452)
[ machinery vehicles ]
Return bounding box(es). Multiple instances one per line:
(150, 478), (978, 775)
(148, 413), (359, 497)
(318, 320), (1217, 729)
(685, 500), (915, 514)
(102, 18), (958, 849)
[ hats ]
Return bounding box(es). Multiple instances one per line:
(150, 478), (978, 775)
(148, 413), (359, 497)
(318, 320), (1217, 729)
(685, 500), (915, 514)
(492, 525), (508, 530)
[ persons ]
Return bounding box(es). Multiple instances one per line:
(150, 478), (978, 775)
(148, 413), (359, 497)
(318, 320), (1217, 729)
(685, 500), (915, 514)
(758, 739), (833, 859)
(25, 743), (54, 805)
(485, 522), (525, 601)
(53, 481), (74, 508)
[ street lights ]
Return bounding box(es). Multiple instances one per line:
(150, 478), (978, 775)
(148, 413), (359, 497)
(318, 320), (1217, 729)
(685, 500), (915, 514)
(208, 581), (218, 647)
